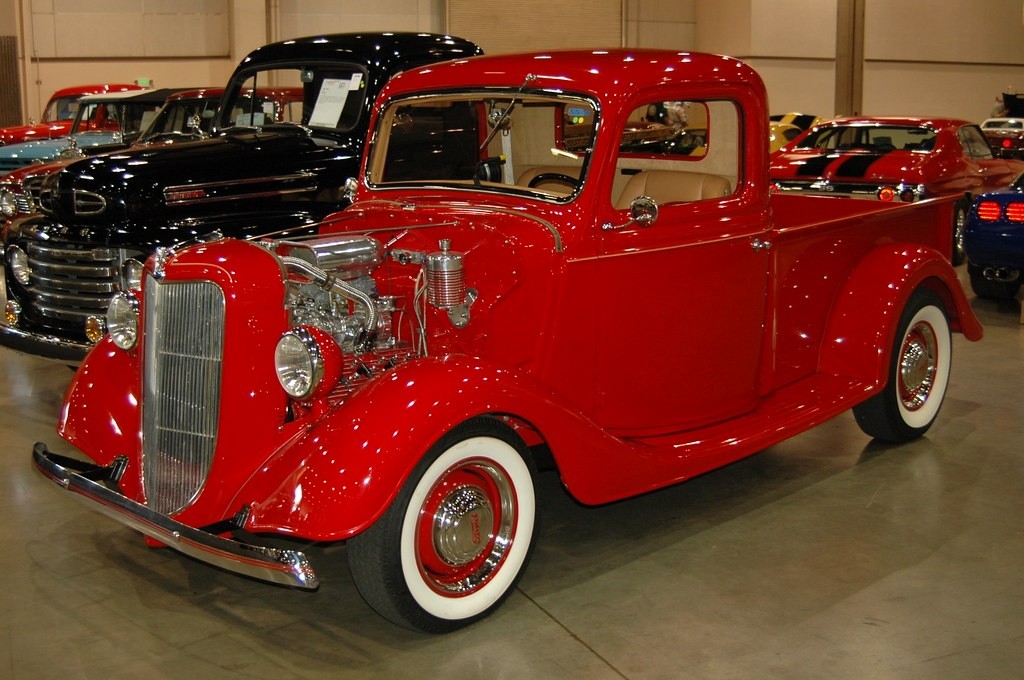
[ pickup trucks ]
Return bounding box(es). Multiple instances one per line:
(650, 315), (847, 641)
(32, 48), (984, 633)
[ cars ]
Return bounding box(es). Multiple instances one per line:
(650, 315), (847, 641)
(964, 173), (1024, 302)
(650, 111), (835, 159)
(0, 87), (225, 178)
(0, 86), (303, 241)
(981, 118), (1024, 160)
(0, 83), (145, 145)
(0, 30), (683, 359)
(768, 115), (1024, 202)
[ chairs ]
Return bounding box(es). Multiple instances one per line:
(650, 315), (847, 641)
(614, 171), (731, 210)
(516, 166), (581, 193)
